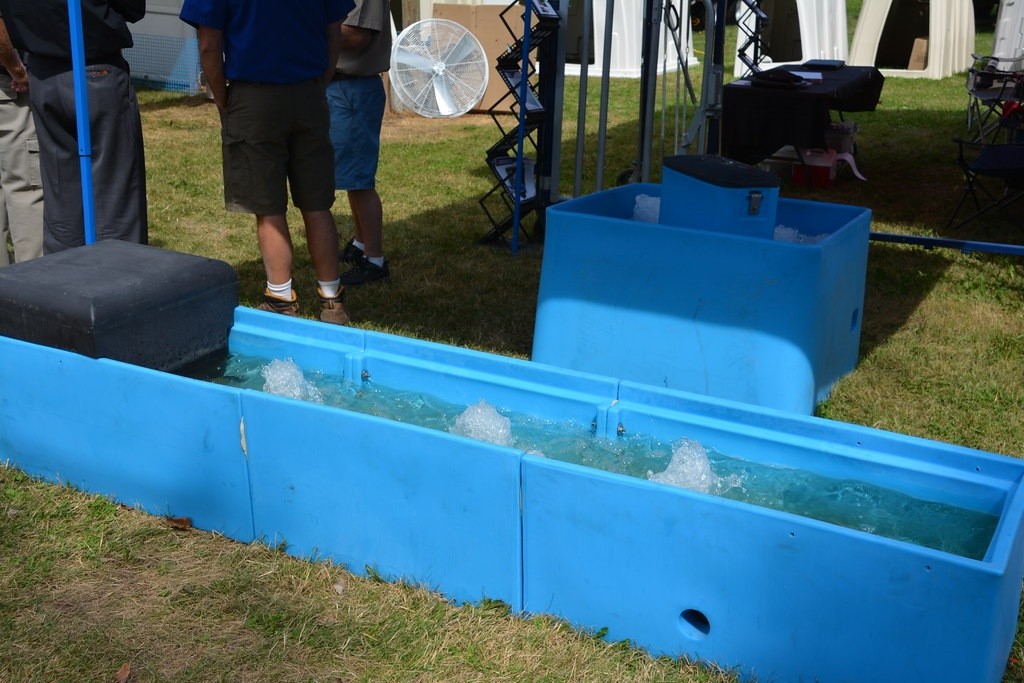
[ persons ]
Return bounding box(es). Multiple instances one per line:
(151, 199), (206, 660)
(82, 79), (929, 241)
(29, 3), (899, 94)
(179, 0), (357, 327)
(324, 0), (394, 287)
(0, 0), (147, 267)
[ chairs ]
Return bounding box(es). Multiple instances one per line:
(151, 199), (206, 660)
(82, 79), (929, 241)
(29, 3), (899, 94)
(946, 55), (1024, 234)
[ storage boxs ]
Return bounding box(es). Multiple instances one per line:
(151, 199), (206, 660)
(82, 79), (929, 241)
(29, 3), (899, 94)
(791, 150), (836, 189)
(432, 3), (539, 113)
(824, 123), (859, 154)
(0, 299), (1024, 683)
(530, 154), (872, 417)
(908, 36), (929, 71)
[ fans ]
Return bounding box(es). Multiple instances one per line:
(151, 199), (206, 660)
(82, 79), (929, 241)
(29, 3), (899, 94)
(387, 19), (489, 119)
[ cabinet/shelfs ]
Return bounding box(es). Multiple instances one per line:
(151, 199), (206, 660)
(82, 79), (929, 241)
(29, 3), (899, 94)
(480, 0), (562, 251)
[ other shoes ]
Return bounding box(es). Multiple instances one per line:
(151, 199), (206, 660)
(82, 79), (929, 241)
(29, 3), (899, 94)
(340, 257), (391, 284)
(338, 236), (364, 261)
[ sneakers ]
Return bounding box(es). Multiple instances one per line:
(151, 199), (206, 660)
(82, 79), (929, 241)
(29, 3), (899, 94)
(316, 284), (359, 324)
(254, 286), (300, 316)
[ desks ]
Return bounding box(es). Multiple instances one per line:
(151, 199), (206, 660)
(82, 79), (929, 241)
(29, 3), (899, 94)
(720, 64), (885, 189)
